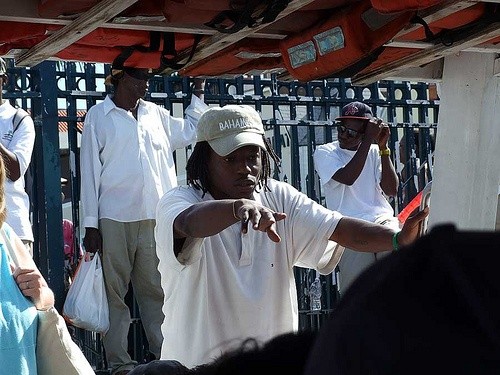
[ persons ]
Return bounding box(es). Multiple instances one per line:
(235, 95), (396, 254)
(81, 66), (209, 375)
(314, 101), (400, 294)
(0, 155), (54, 375)
(0, 58), (34, 256)
(154, 105), (429, 369)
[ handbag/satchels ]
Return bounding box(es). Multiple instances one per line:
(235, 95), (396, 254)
(62, 252), (111, 334)
(36, 306), (98, 375)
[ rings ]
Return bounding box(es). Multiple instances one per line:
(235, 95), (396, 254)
(26, 282), (30, 289)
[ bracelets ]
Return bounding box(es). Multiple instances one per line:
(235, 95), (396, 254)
(393, 231), (400, 250)
(233, 200), (240, 220)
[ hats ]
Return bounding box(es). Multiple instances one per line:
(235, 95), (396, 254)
(195, 105), (268, 156)
(0, 58), (7, 76)
(103, 50), (155, 86)
(335, 101), (373, 121)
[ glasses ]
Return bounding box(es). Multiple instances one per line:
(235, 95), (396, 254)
(337, 124), (363, 139)
